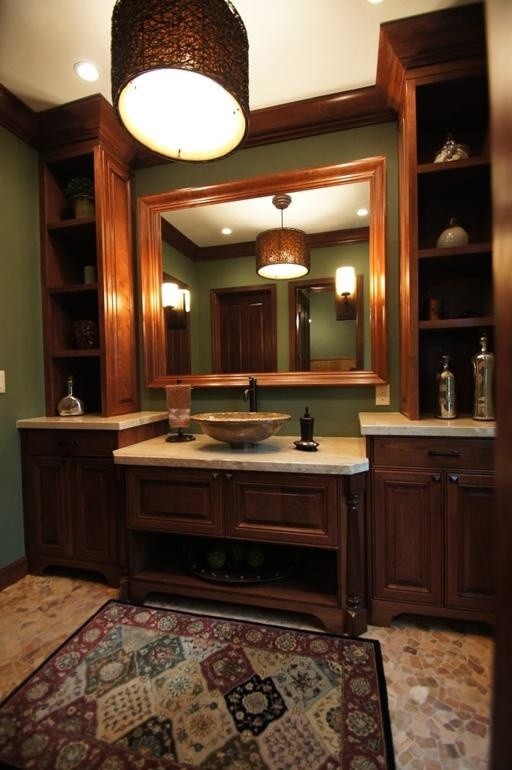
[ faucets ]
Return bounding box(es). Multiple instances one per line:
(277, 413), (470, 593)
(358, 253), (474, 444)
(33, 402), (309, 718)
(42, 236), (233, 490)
(243, 377), (259, 412)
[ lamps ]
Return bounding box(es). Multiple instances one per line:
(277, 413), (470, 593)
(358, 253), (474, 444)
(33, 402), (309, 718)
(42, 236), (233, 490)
(109, 0), (251, 163)
(254, 193), (312, 281)
(163, 281), (178, 322)
(334, 264), (364, 310)
(176, 288), (191, 330)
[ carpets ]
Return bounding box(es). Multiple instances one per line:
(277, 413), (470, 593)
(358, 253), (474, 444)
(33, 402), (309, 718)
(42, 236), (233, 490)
(0, 595), (397, 769)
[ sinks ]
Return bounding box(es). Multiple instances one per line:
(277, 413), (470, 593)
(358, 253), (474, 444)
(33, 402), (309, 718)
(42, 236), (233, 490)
(190, 411), (291, 447)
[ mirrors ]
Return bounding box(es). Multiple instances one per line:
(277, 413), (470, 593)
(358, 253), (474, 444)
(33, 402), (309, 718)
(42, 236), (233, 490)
(135, 155), (391, 389)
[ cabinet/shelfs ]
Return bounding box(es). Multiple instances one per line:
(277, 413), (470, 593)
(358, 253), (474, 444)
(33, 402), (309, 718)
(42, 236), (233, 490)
(36, 138), (141, 419)
(126, 466), (370, 639)
(358, 407), (498, 627)
(397, 55), (497, 422)
(16, 410), (169, 568)
